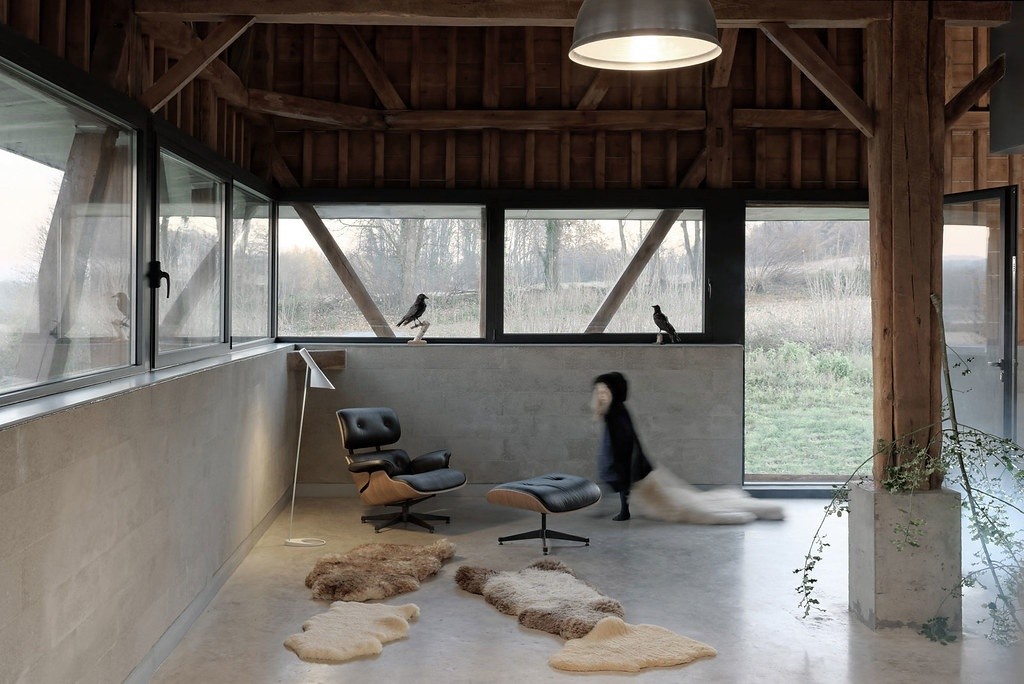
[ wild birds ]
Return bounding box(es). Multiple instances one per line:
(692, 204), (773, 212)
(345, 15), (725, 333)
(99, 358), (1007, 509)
(396, 294), (429, 328)
(651, 305), (681, 343)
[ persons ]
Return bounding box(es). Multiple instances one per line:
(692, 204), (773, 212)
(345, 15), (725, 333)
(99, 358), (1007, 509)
(591, 371), (657, 521)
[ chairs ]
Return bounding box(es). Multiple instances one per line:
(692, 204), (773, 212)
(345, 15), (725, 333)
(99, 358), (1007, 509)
(336, 408), (469, 533)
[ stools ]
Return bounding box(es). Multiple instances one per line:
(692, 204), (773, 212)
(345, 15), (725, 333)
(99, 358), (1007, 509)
(486, 474), (601, 556)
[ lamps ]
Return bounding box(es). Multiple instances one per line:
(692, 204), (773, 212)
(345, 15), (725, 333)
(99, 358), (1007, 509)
(285, 347), (336, 547)
(568, 0), (722, 71)
